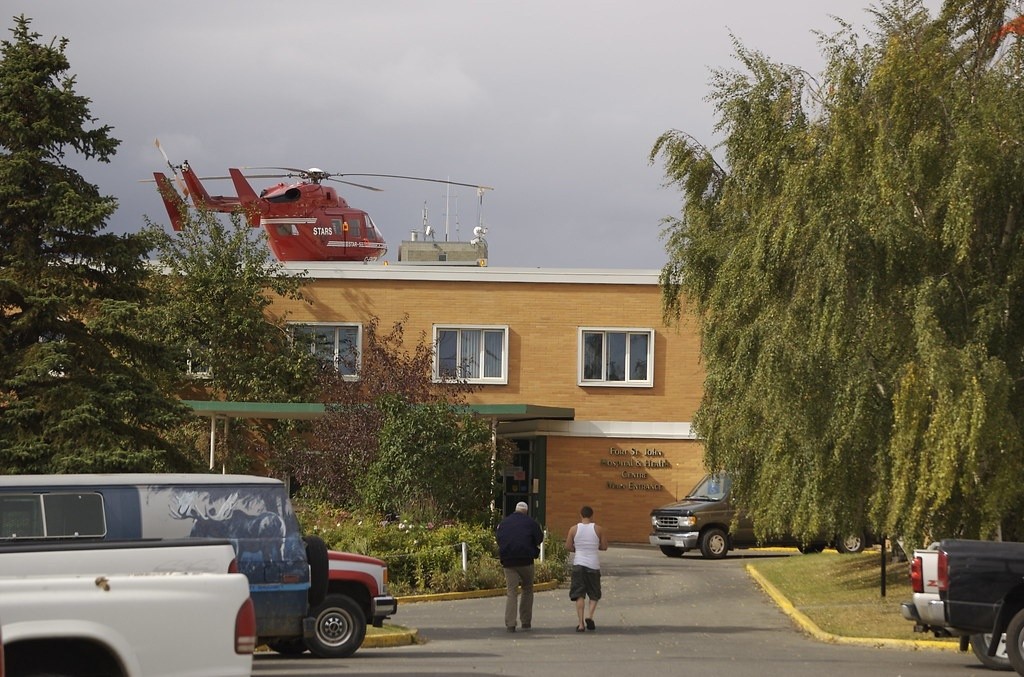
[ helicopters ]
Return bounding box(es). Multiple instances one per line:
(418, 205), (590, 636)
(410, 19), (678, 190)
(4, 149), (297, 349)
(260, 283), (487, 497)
(134, 136), (494, 261)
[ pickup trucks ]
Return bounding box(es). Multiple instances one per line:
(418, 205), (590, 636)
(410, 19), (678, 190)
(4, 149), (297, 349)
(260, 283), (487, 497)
(0, 534), (256, 677)
(900, 536), (1024, 677)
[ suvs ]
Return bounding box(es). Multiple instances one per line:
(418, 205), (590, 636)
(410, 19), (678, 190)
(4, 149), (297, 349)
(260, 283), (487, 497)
(0, 470), (330, 660)
(270, 550), (400, 659)
(649, 469), (881, 560)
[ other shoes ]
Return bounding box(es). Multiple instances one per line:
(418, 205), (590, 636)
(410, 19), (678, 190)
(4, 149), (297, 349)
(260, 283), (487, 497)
(521, 624), (531, 628)
(507, 626), (515, 632)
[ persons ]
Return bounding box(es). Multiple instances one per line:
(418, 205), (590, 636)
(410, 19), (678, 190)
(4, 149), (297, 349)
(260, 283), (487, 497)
(564, 506), (608, 631)
(496, 501), (544, 634)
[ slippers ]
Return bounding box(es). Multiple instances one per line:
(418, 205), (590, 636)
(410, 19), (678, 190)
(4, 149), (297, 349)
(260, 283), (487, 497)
(585, 618), (595, 631)
(576, 625), (585, 632)
